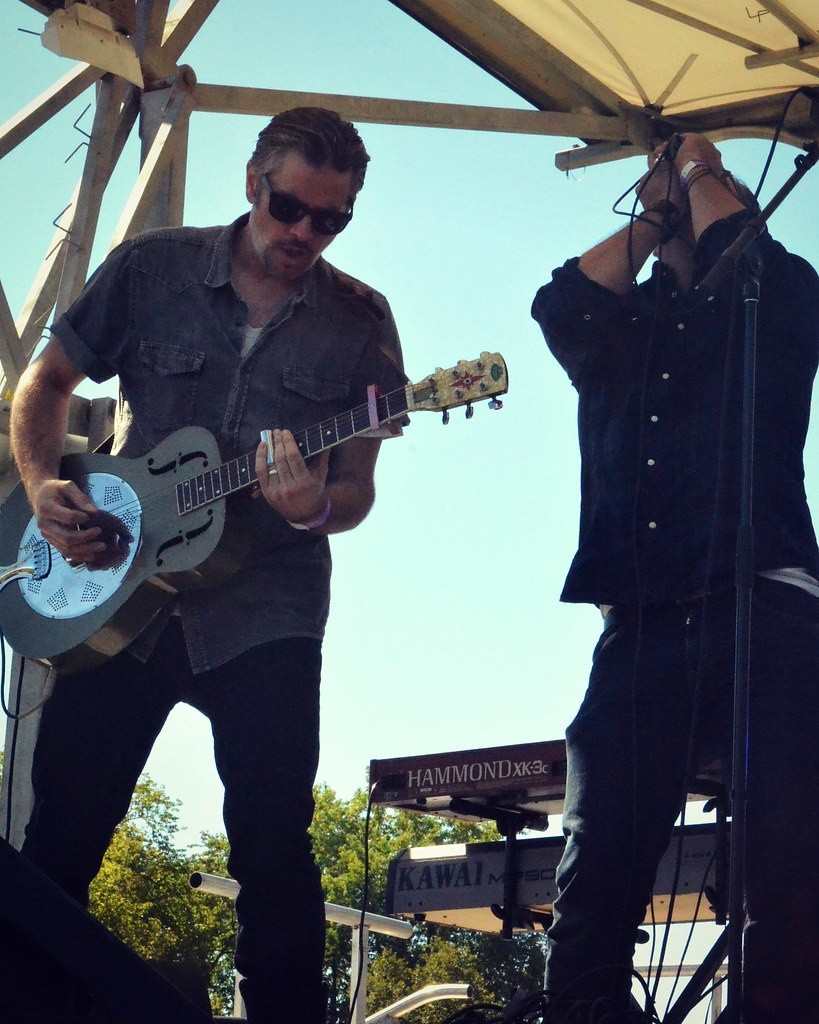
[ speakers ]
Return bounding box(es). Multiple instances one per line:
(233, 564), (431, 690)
(0, 837), (210, 1023)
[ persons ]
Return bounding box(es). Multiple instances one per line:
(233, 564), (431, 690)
(10, 108), (410, 1024)
(531, 133), (819, 1024)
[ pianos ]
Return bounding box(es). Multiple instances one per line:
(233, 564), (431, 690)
(366, 737), (718, 824)
(382, 821), (729, 938)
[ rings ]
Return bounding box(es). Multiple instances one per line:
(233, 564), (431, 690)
(268, 469), (277, 475)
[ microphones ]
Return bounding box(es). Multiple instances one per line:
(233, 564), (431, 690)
(665, 133), (684, 159)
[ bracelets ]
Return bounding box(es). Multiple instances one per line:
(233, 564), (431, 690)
(645, 199), (684, 244)
(286, 496), (331, 529)
(679, 159), (715, 192)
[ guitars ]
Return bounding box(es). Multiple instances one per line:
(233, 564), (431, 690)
(0, 349), (510, 678)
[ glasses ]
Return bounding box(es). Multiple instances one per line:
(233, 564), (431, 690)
(260, 173), (354, 235)
